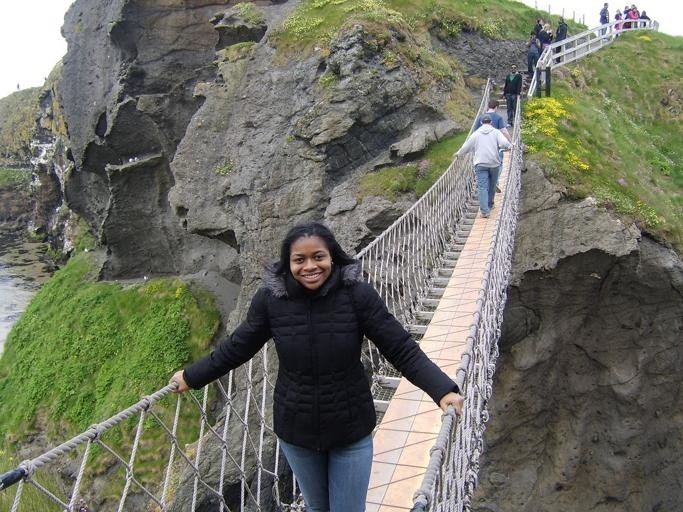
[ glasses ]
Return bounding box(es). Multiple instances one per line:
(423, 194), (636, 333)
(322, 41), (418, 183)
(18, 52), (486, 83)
(511, 67), (516, 69)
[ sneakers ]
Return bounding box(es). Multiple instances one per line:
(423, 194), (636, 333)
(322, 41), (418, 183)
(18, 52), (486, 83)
(496, 184), (502, 194)
(482, 214), (492, 221)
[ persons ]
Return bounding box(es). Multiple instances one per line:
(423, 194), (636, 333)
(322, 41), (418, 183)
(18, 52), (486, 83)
(169, 219), (467, 510)
(554, 16), (567, 64)
(524, 31), (541, 76)
(451, 114), (514, 219)
(599, 2), (610, 42)
(538, 23), (554, 65)
(472, 100), (513, 193)
(499, 63), (522, 128)
(534, 18), (544, 39)
(613, 4), (652, 37)
